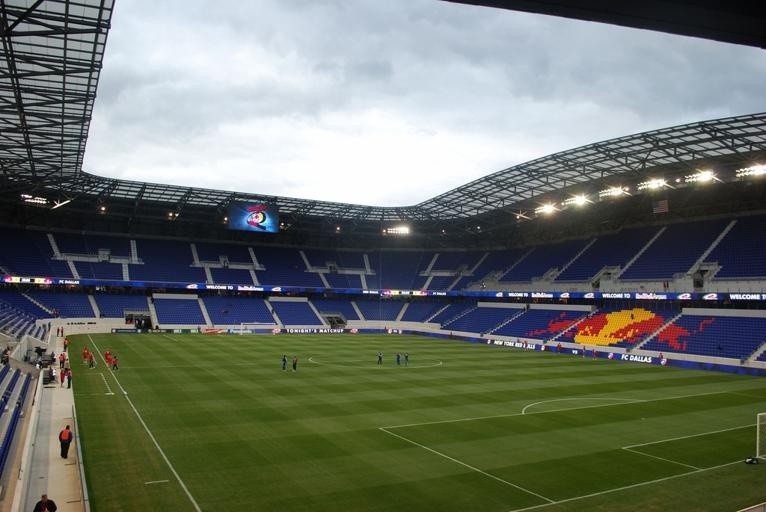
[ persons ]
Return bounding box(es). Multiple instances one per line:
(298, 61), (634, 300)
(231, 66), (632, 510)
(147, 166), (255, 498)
(58, 424), (72, 459)
(292, 356), (297, 372)
(403, 352), (407, 367)
(591, 348), (596, 361)
(582, 345), (586, 359)
(0, 321), (118, 389)
(376, 350), (383, 365)
(125, 315), (151, 334)
(280, 354), (287, 373)
(32, 494), (57, 512)
(556, 342), (561, 353)
(524, 339), (528, 352)
(395, 352), (401, 366)
(658, 352), (663, 358)
